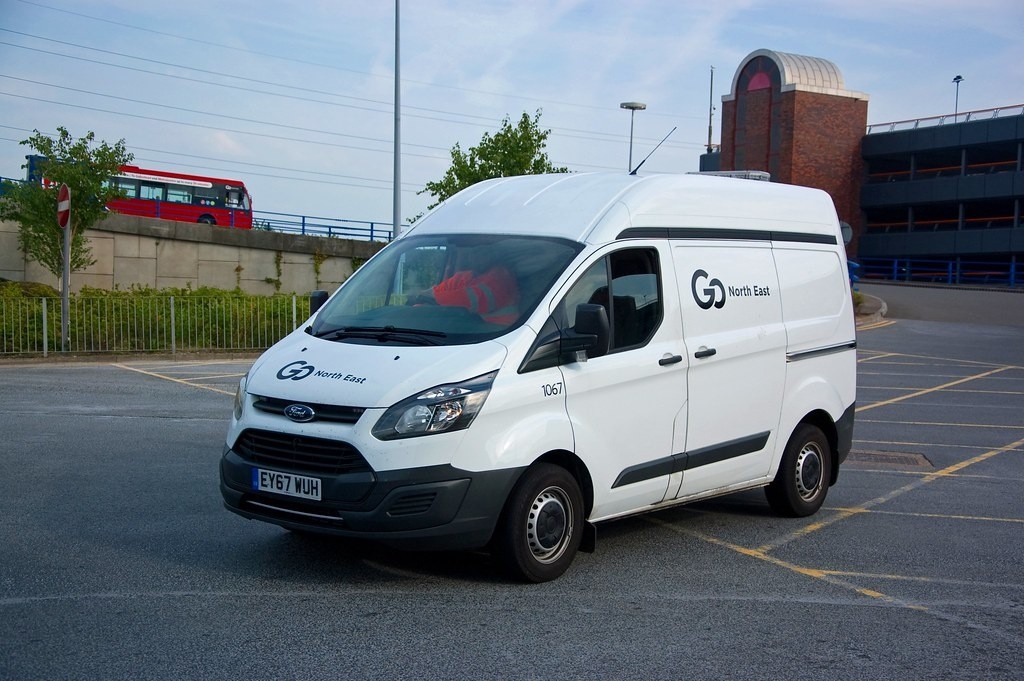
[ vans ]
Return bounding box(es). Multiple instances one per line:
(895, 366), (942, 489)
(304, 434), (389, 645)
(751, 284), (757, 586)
(221, 172), (856, 584)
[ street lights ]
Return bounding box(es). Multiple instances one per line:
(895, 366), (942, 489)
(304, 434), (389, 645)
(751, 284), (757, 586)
(620, 102), (646, 172)
(952, 74), (965, 123)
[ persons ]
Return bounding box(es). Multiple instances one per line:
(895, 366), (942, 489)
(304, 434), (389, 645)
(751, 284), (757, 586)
(415, 252), (518, 325)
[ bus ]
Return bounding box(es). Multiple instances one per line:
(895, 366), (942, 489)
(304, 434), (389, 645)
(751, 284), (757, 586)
(21, 155), (253, 231)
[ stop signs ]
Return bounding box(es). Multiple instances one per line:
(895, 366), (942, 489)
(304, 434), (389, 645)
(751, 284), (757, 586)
(58, 182), (69, 228)
